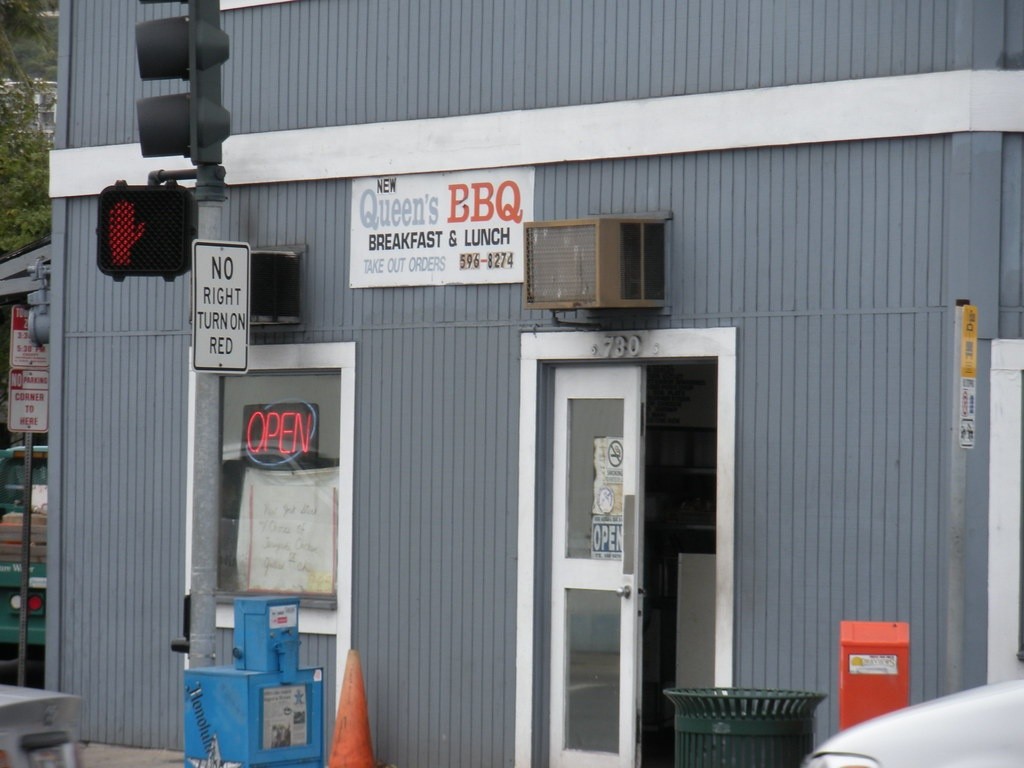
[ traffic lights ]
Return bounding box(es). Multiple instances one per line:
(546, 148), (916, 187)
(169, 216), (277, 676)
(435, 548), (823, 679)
(133, 0), (195, 164)
(97, 184), (192, 281)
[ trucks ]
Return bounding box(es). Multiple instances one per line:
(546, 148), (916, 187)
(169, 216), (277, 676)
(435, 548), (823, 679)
(1, 444), (49, 647)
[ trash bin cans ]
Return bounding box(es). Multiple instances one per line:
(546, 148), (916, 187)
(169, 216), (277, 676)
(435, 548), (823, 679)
(663, 687), (829, 768)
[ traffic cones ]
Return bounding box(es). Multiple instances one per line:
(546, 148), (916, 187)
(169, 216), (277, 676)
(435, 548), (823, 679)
(322, 647), (377, 768)
